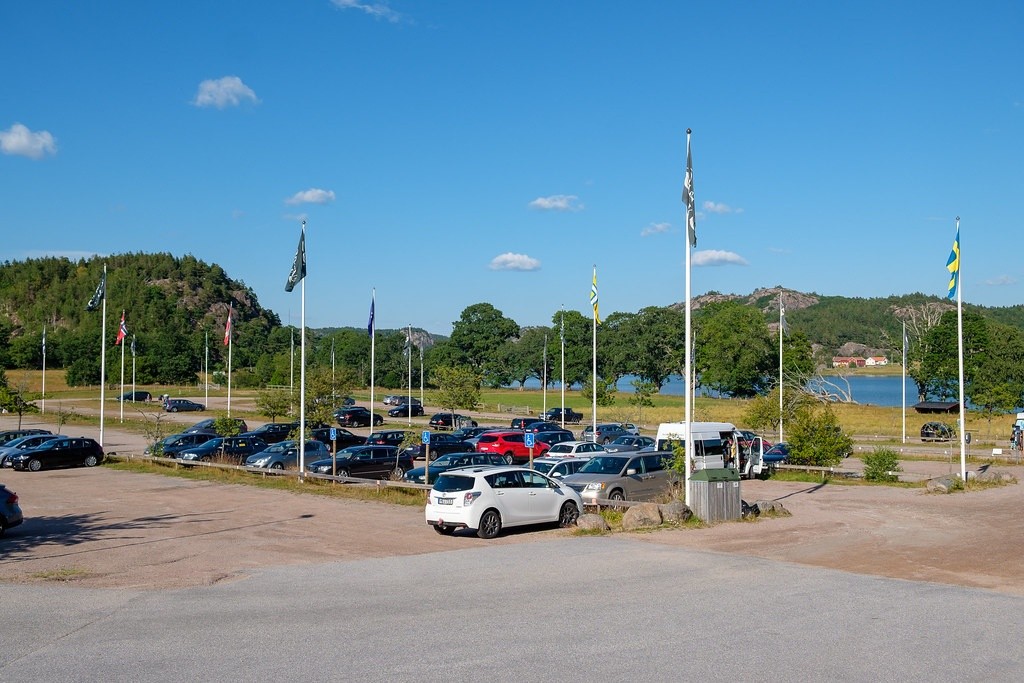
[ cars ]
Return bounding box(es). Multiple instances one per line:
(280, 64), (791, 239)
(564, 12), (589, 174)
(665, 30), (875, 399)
(0, 483), (24, 539)
(425, 466), (584, 539)
(163, 398), (205, 413)
(10, 437), (104, 472)
(0, 434), (72, 469)
(143, 394), (657, 489)
(0, 428), (52, 446)
(728, 430), (809, 465)
(920, 422), (957, 442)
(117, 391), (152, 402)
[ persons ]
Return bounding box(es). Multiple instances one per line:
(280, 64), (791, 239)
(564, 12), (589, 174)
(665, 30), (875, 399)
(158, 394), (171, 410)
(634, 433), (640, 437)
(602, 436), (611, 445)
(1010, 424), (1024, 455)
(722, 436), (736, 468)
(147, 393), (150, 400)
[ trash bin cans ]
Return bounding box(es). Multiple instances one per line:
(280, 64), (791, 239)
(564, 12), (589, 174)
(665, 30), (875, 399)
(688, 469), (741, 521)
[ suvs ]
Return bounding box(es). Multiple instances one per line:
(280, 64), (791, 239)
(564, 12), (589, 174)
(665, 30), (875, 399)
(563, 451), (685, 512)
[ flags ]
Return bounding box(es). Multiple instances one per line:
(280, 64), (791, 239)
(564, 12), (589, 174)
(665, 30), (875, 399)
(290, 329), (295, 346)
(41, 328), (46, 354)
(904, 329), (909, 368)
(129, 339), (135, 355)
(946, 226), (960, 298)
(367, 295), (375, 337)
(402, 327), (412, 355)
(329, 341), (334, 363)
(780, 301), (791, 341)
(544, 339), (549, 360)
(681, 142), (696, 246)
(591, 273), (601, 325)
(223, 306), (231, 345)
(690, 342), (694, 362)
(115, 312), (128, 345)
(285, 228), (307, 292)
(559, 312), (567, 347)
(419, 341), (425, 361)
(86, 273), (105, 312)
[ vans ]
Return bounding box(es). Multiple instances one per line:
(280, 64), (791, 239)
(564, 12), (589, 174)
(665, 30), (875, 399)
(654, 421), (763, 479)
